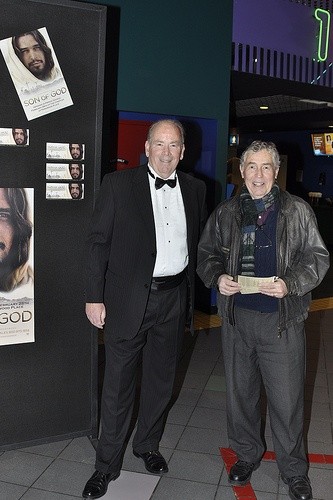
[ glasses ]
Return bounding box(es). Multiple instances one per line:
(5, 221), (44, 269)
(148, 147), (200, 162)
(244, 222), (272, 248)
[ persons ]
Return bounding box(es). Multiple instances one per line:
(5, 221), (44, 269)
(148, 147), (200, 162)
(0, 30), (83, 293)
(81, 118), (206, 500)
(196, 141), (330, 500)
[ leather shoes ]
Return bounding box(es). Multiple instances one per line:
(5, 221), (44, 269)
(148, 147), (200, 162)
(132, 448), (168, 474)
(228, 459), (260, 486)
(281, 473), (312, 500)
(82, 469), (121, 500)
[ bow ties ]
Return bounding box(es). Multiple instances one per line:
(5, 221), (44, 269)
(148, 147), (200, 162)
(147, 165), (177, 190)
